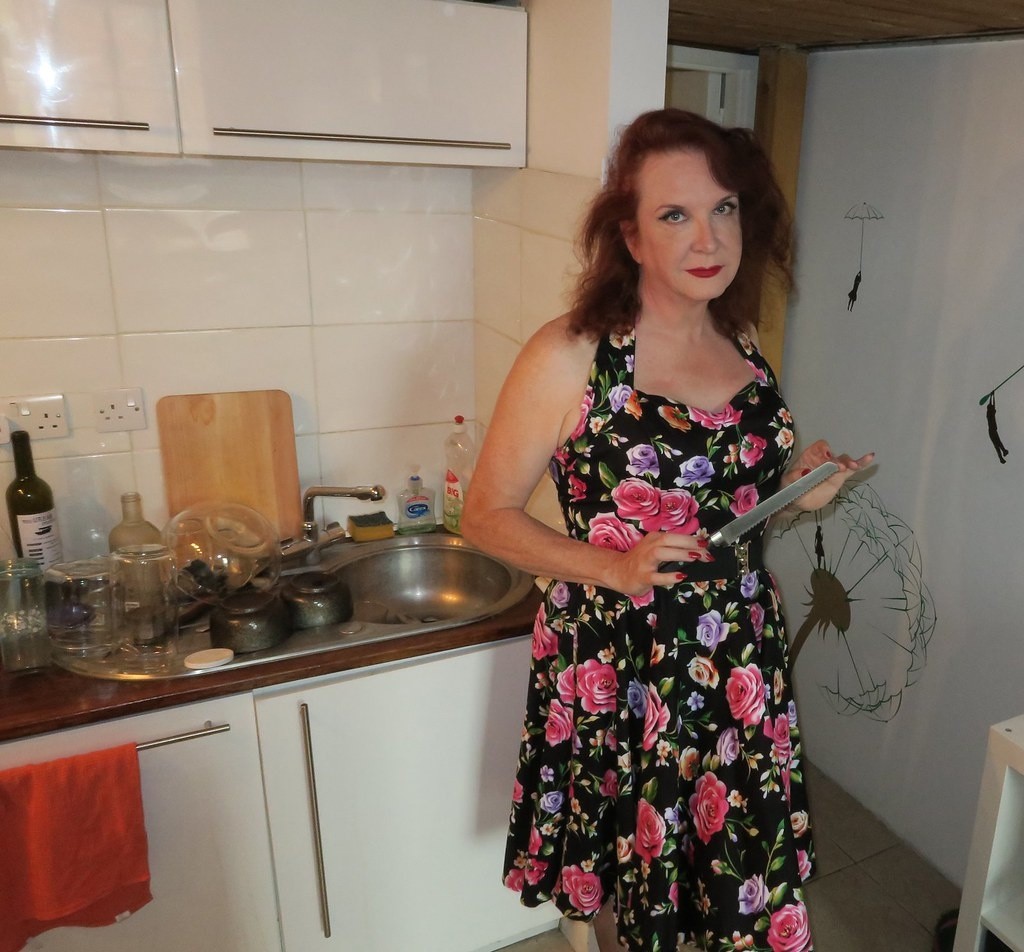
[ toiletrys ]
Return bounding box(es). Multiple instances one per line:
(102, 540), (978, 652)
(394, 464), (437, 535)
(442, 415), (476, 535)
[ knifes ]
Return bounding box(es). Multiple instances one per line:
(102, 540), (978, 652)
(658, 460), (841, 578)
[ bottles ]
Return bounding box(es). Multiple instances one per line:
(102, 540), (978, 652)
(107, 492), (167, 646)
(5, 431), (65, 566)
(442, 415), (477, 536)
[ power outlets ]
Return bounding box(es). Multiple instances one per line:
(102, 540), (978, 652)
(0, 394), (68, 444)
(93, 386), (145, 433)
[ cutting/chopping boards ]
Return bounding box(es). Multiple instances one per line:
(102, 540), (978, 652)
(153, 389), (305, 570)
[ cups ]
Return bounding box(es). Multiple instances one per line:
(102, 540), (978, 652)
(42, 560), (115, 660)
(110, 544), (178, 662)
(0, 558), (54, 674)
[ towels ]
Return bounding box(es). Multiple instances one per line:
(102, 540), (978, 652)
(2, 743), (152, 952)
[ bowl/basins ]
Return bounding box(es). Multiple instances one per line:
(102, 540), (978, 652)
(209, 591), (292, 654)
(160, 500), (284, 608)
(280, 570), (354, 627)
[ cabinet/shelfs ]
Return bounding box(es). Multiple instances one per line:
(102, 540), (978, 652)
(952, 712), (1023, 951)
(250, 632), (561, 952)
(0, 685), (282, 951)
(169, 0), (527, 168)
(0, 1), (183, 158)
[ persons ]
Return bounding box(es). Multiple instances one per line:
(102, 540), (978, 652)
(460, 107), (875, 952)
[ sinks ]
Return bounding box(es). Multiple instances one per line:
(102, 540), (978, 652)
(328, 547), (512, 624)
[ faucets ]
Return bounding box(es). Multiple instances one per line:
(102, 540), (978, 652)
(301, 485), (386, 544)
